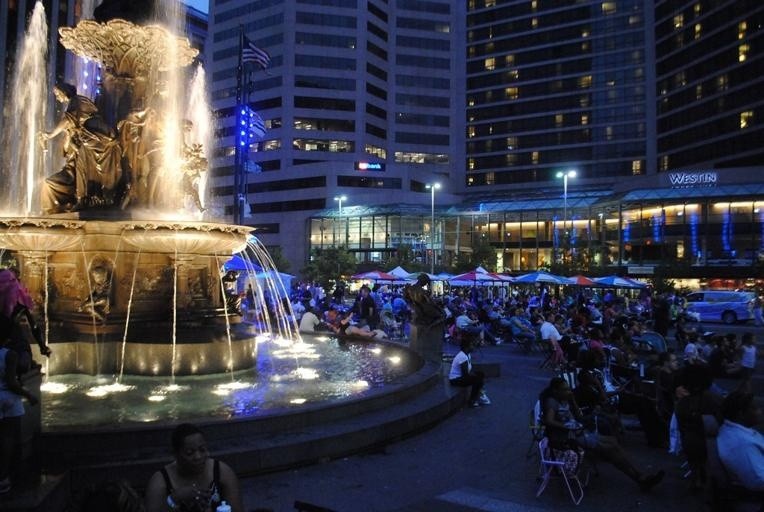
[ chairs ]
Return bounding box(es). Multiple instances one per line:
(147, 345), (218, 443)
(509, 327), (732, 511)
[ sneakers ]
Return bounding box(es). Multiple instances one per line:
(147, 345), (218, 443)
(0, 476), (14, 493)
(639, 469), (665, 493)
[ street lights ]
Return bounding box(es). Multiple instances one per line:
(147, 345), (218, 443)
(555, 170), (576, 229)
(334, 196), (347, 244)
(425, 182), (441, 233)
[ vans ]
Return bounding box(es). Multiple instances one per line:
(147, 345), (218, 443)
(685, 290), (757, 325)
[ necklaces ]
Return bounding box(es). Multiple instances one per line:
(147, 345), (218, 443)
(172, 461), (208, 488)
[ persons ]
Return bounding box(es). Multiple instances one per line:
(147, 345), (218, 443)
(217, 269), (245, 318)
(402, 272), (448, 326)
(231, 285), (537, 357)
(447, 336), (493, 408)
(76, 266), (112, 325)
(36, 81), (209, 218)
(71, 477), (146, 512)
(145, 421), (246, 511)
(0, 265), (52, 492)
(539, 288), (764, 512)
